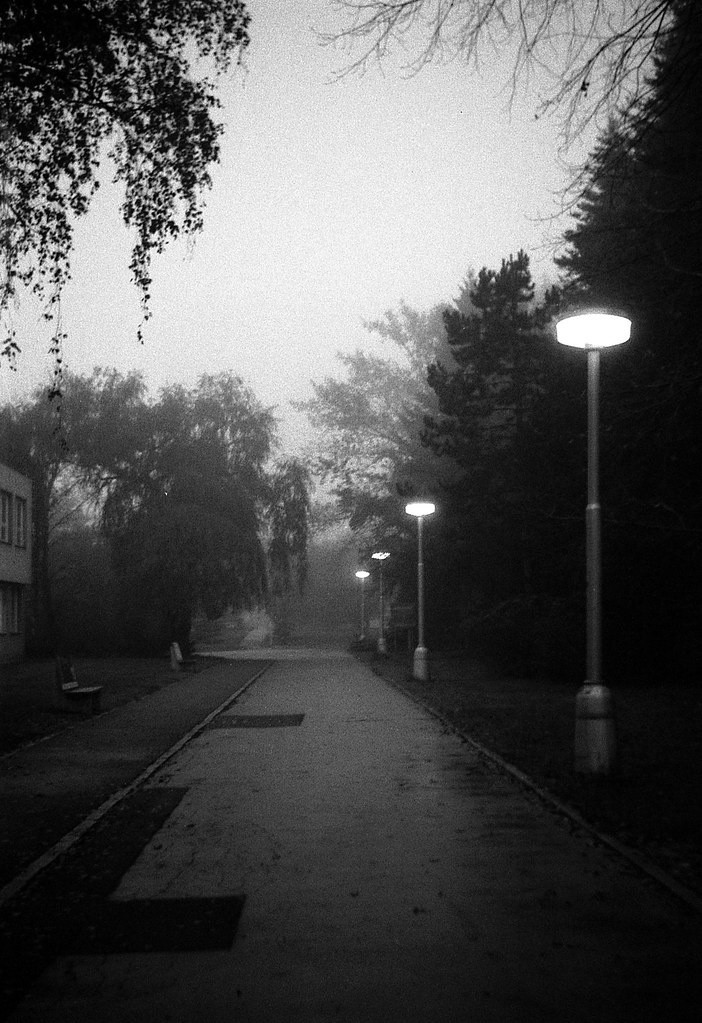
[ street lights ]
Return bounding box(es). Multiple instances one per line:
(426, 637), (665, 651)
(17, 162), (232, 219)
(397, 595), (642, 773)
(552, 305), (635, 785)
(371, 549), (392, 656)
(354, 568), (371, 641)
(403, 500), (437, 684)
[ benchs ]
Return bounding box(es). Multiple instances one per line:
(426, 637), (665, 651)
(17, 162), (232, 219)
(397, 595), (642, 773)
(171, 641), (198, 673)
(53, 655), (105, 717)
